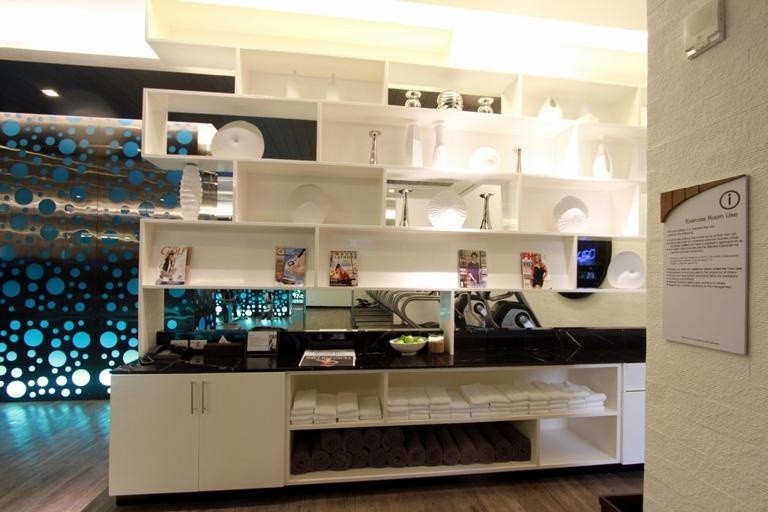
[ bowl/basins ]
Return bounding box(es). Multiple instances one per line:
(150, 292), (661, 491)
(389, 337), (428, 356)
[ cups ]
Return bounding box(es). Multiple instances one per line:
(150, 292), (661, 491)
(429, 335), (444, 355)
(477, 97), (494, 113)
(404, 90), (422, 107)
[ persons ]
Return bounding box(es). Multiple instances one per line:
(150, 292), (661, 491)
(284, 260), (294, 276)
(532, 255), (547, 288)
(160, 250), (175, 280)
(335, 264), (349, 280)
(467, 253), (480, 284)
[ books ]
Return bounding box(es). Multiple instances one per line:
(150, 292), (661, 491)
(351, 307), (401, 329)
(457, 249), (487, 288)
(330, 251), (358, 286)
(273, 248), (306, 286)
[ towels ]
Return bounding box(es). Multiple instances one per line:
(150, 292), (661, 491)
(293, 431), (527, 473)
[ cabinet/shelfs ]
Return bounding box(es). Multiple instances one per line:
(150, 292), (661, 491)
(108, 3), (645, 508)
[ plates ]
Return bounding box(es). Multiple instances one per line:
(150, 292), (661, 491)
(607, 251), (645, 289)
(286, 184), (330, 224)
(551, 195), (589, 234)
(210, 120), (265, 160)
(426, 190), (468, 228)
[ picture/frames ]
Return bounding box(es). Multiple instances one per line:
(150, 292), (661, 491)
(455, 247), (489, 288)
(270, 245), (310, 286)
(659, 172), (752, 355)
(324, 249), (358, 286)
